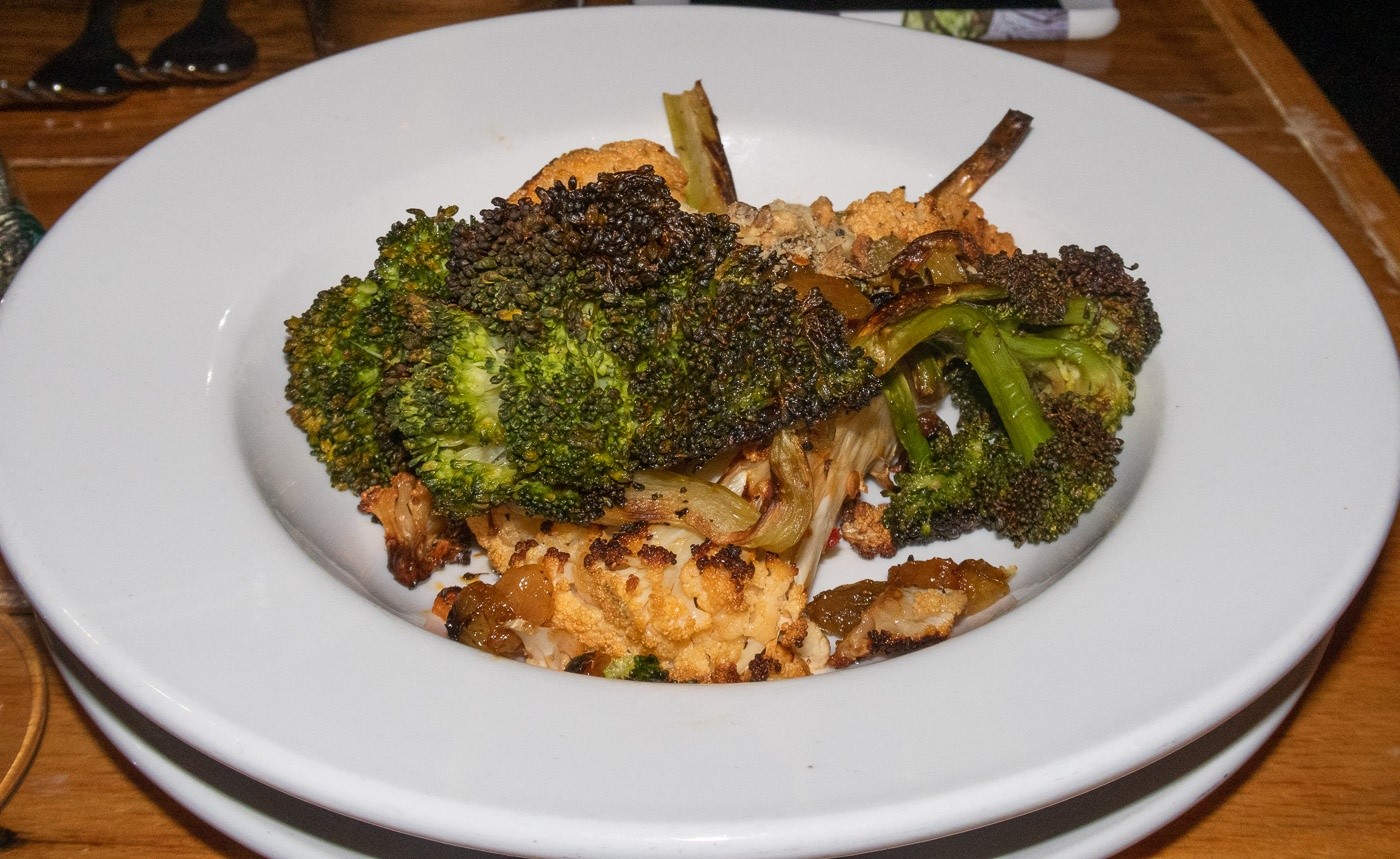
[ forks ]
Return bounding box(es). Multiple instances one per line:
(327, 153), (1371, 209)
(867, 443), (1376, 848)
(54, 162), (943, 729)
(117, 0), (260, 84)
(2, 0), (141, 105)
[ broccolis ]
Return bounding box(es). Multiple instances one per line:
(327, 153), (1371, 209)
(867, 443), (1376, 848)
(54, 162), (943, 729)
(284, 136), (1162, 682)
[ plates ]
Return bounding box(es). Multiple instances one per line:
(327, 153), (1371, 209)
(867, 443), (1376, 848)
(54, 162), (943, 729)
(1, 6), (1398, 858)
(32, 612), (1338, 858)
